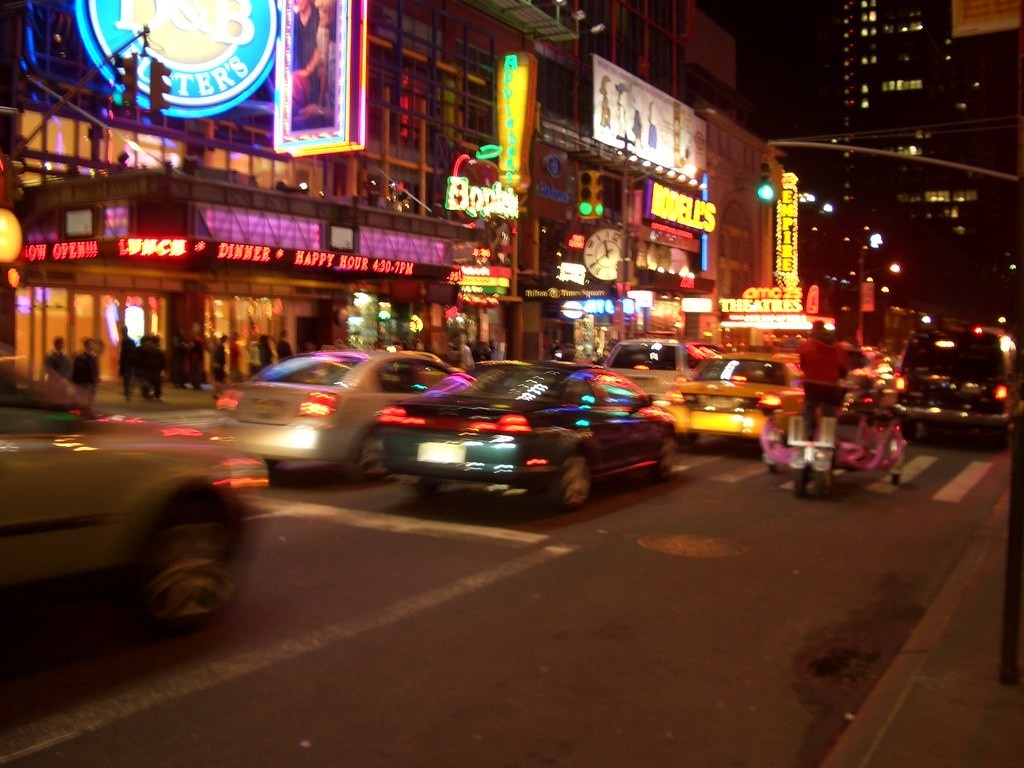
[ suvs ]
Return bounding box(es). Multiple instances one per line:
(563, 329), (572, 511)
(892, 322), (1021, 447)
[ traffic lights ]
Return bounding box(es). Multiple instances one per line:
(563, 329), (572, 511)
(576, 168), (606, 221)
(148, 58), (174, 113)
(113, 56), (140, 110)
(757, 155), (783, 205)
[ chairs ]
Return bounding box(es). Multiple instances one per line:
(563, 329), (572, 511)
(396, 366), (420, 392)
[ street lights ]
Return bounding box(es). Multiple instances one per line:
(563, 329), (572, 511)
(853, 248), (904, 348)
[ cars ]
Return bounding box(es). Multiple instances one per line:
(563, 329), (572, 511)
(213, 341), (469, 486)
(664, 349), (804, 453)
(373, 357), (681, 514)
(602, 336), (729, 397)
(0, 342), (269, 639)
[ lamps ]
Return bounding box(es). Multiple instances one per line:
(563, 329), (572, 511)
(534, 23), (605, 40)
(531, 10), (586, 30)
(501, 0), (567, 15)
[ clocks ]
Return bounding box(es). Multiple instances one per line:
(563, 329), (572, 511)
(583, 225), (623, 282)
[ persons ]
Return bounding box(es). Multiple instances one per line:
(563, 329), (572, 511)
(755, 315), (938, 447)
(290, 0), (337, 130)
(42, 311), (634, 419)
